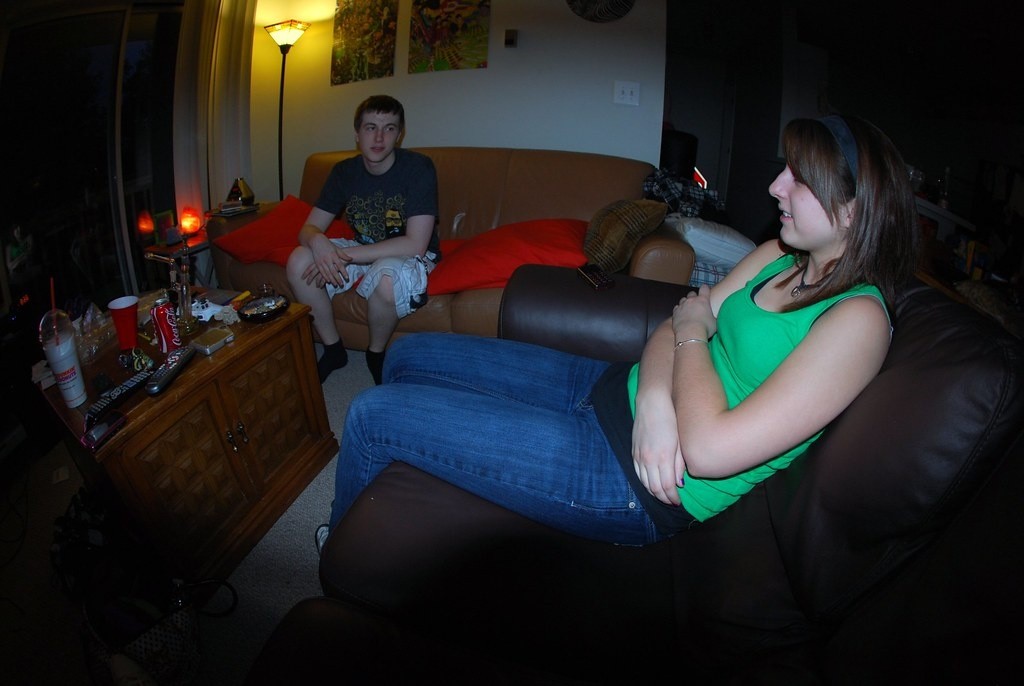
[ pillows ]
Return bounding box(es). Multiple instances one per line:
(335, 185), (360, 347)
(211, 193), (365, 294)
(425, 218), (590, 297)
(583, 198), (668, 279)
(665, 217), (757, 272)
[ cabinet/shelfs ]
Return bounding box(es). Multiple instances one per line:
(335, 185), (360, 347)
(31, 282), (340, 607)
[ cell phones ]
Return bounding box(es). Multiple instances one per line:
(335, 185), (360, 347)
(578, 264), (615, 289)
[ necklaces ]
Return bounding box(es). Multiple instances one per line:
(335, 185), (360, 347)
(789, 262), (828, 298)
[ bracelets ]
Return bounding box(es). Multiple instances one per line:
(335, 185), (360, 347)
(674, 338), (712, 354)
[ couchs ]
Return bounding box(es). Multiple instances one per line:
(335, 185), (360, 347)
(242, 262), (1024, 686)
(205, 146), (696, 356)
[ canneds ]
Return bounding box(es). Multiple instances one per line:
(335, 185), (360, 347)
(151, 298), (183, 353)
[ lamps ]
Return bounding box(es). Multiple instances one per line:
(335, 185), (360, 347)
(264, 19), (313, 204)
(137, 206), (205, 338)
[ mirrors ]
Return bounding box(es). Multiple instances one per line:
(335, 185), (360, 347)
(0, 0), (140, 317)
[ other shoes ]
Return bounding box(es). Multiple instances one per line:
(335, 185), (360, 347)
(315, 524), (329, 554)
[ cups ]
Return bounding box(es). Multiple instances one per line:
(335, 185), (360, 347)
(40, 309), (87, 408)
(257, 281), (273, 297)
(108, 296), (138, 350)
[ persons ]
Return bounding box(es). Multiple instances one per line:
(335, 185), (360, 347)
(312, 108), (920, 614)
(288, 94), (441, 386)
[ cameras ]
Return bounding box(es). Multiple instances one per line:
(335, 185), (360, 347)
(190, 327), (234, 355)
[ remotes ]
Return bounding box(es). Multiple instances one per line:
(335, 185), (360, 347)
(87, 369), (156, 420)
(145, 346), (197, 395)
